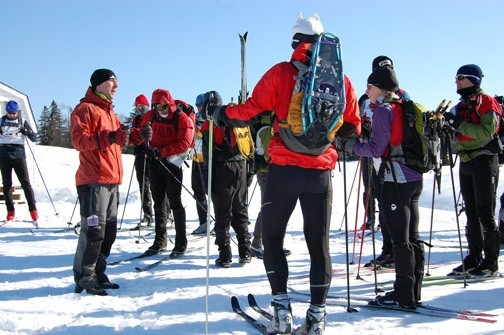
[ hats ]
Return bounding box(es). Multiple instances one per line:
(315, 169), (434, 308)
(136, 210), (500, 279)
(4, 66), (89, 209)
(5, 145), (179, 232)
(456, 63), (481, 87)
(372, 57), (393, 71)
(368, 66), (399, 93)
(90, 69), (117, 87)
(135, 94), (149, 107)
(195, 94), (206, 106)
(291, 12), (324, 49)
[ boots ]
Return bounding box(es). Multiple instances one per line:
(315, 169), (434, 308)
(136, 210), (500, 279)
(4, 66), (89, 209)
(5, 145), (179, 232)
(265, 299), (292, 334)
(294, 311), (326, 334)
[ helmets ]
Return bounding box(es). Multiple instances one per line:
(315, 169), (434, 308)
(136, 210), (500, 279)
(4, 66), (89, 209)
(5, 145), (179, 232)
(5, 101), (18, 112)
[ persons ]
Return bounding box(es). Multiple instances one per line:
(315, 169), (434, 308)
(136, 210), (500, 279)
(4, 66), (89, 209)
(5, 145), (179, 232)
(0, 100), (39, 221)
(207, 11), (362, 335)
(129, 95), (174, 228)
(201, 91), (251, 265)
(70, 69), (153, 295)
(192, 94), (219, 236)
(367, 205), (395, 268)
(251, 109), (291, 259)
(335, 68), (432, 309)
(359, 56), (395, 229)
(140, 89), (195, 258)
(443, 64), (503, 278)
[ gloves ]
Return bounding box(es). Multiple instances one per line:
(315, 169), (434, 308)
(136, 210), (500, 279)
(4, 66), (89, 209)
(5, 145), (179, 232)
(335, 132), (356, 156)
(131, 122), (152, 146)
(96, 124), (129, 150)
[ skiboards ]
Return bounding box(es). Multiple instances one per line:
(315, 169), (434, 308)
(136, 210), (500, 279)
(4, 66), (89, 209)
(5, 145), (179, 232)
(332, 260), (447, 278)
(358, 271), (504, 292)
(106, 246), (204, 271)
(231, 294), (273, 335)
(329, 229), (378, 239)
(117, 226), (156, 238)
(0, 221), (39, 229)
(287, 287), (498, 322)
(141, 233), (211, 244)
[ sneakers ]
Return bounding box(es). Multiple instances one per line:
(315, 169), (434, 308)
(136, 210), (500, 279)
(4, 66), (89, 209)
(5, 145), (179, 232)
(76, 284), (107, 295)
(172, 246), (185, 253)
(149, 245), (167, 252)
(193, 222), (207, 233)
(377, 290), (422, 309)
(373, 252), (395, 269)
(100, 281), (120, 289)
(468, 260), (498, 277)
(452, 256), (482, 274)
(139, 218), (153, 227)
(215, 256), (232, 267)
(238, 253), (251, 263)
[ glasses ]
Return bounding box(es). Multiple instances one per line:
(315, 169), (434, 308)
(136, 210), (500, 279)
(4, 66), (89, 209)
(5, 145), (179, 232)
(455, 76), (468, 80)
(367, 84), (380, 90)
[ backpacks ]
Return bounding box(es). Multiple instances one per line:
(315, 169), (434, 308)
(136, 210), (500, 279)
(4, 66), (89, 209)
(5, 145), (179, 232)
(381, 97), (434, 172)
(278, 32), (346, 155)
(153, 100), (197, 148)
